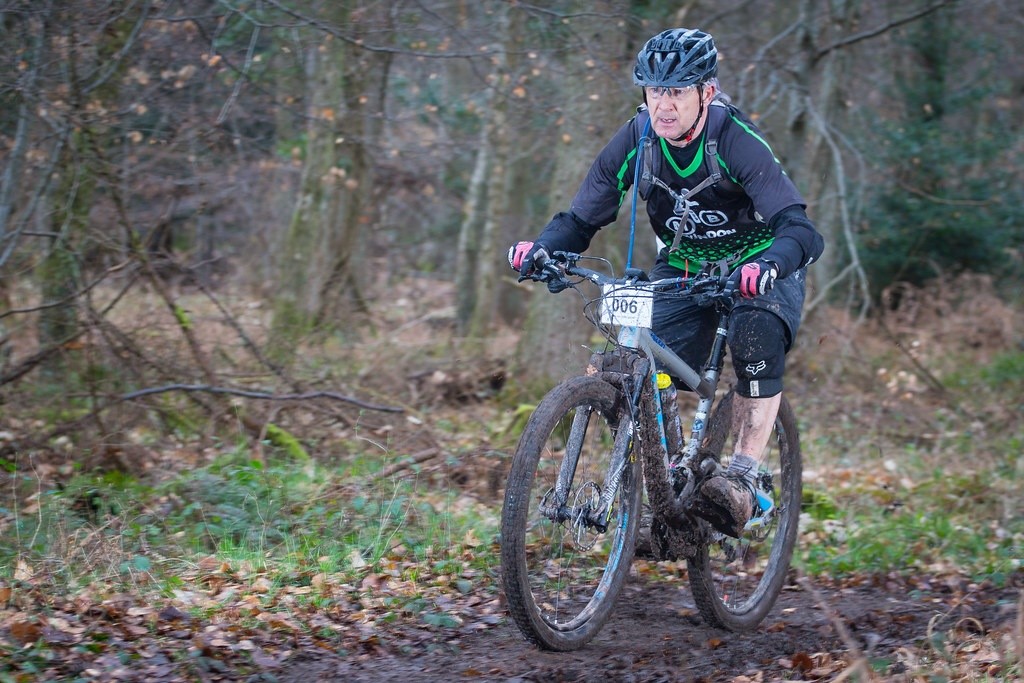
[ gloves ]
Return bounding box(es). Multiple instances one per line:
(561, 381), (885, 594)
(723, 261), (778, 305)
(507, 241), (550, 282)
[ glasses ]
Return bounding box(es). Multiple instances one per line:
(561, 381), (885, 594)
(642, 83), (699, 98)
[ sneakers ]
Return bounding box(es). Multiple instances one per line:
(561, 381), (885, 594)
(633, 502), (653, 555)
(700, 467), (757, 539)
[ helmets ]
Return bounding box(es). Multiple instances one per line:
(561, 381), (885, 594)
(632, 28), (718, 88)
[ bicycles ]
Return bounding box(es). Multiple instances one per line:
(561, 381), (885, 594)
(498, 249), (807, 653)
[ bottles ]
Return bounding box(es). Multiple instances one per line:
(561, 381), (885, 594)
(656, 369), (684, 454)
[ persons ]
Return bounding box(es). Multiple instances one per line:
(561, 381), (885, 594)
(508, 28), (825, 538)
